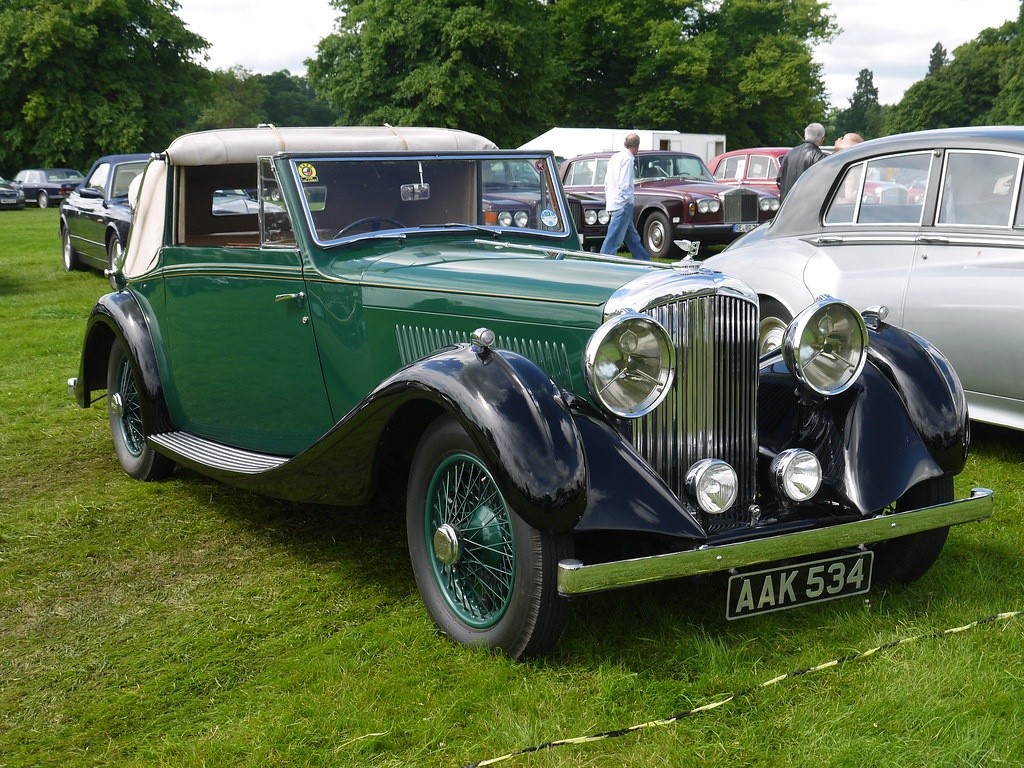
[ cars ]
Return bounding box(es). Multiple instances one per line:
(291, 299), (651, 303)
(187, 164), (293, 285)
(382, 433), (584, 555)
(65, 124), (994, 663)
(559, 150), (781, 259)
(12, 169), (86, 209)
(705, 147), (926, 206)
(480, 158), (586, 246)
(0, 177), (26, 210)
(60, 153), (286, 292)
(698, 125), (1024, 433)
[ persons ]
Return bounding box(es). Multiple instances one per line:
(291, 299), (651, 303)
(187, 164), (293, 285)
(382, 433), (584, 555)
(776, 123), (866, 207)
(599, 133), (651, 261)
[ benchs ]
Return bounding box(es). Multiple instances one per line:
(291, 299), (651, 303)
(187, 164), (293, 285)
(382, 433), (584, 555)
(185, 229), (336, 249)
